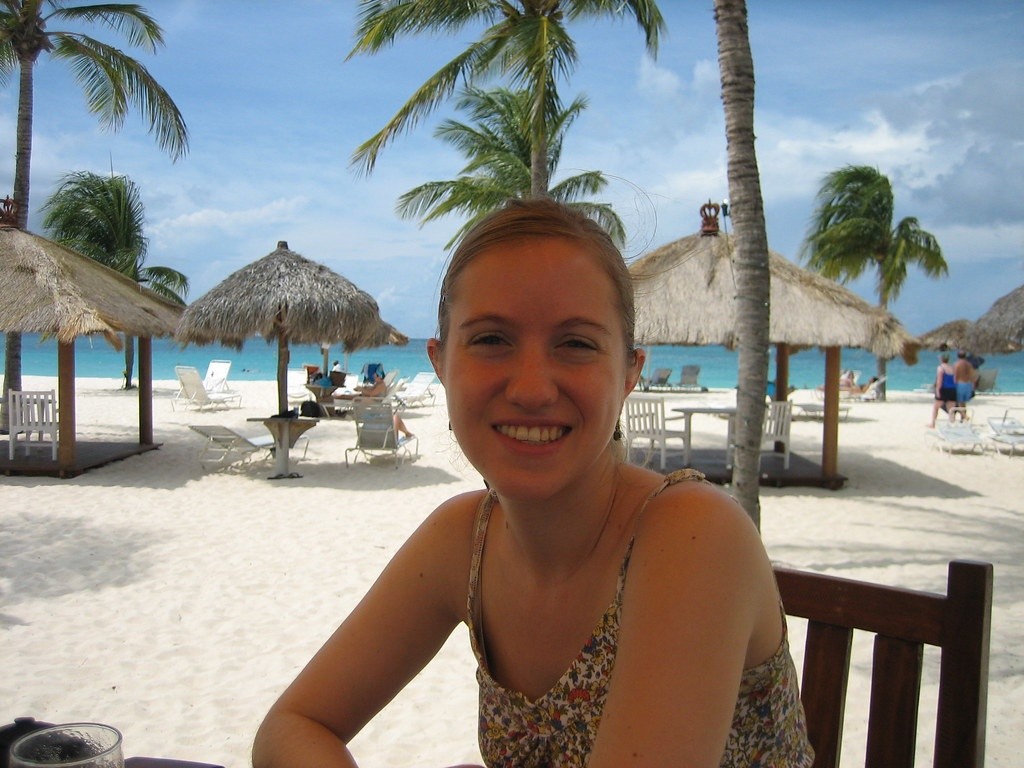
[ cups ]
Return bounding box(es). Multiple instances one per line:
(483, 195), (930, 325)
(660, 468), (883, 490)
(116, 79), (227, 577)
(8, 722), (125, 768)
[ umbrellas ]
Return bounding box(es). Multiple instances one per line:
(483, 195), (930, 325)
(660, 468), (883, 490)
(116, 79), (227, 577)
(921, 284), (1024, 360)
(175, 241), (408, 480)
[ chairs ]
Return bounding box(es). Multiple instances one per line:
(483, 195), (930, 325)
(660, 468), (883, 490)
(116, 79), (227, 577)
(793, 401), (851, 423)
(171, 359), (232, 397)
(640, 368), (672, 392)
(382, 368), (399, 387)
(345, 397), (420, 470)
(924, 406), (987, 456)
(814, 369), (862, 399)
(188, 423), (311, 473)
(854, 375), (888, 402)
(288, 392), (310, 406)
(171, 366), (242, 413)
(397, 372), (435, 407)
(976, 367), (1001, 396)
(673, 365), (701, 392)
(726, 400), (793, 470)
(625, 394), (690, 469)
(344, 374), (358, 386)
(9, 388), (60, 460)
(986, 407), (1024, 459)
(335, 377), (406, 413)
(774, 559), (994, 768)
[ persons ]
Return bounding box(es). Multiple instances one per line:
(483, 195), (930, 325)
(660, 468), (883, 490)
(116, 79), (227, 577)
(952, 351), (978, 423)
(926, 354), (956, 428)
(354, 369), (386, 397)
(250, 202), (814, 767)
(332, 360), (341, 372)
(392, 415), (413, 444)
(840, 370), (878, 393)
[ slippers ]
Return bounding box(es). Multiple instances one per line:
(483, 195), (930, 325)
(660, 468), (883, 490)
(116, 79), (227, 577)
(285, 472), (303, 478)
(267, 473), (285, 479)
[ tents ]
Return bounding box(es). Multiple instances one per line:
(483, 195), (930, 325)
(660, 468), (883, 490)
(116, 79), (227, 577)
(0, 222), (187, 478)
(627, 203), (907, 481)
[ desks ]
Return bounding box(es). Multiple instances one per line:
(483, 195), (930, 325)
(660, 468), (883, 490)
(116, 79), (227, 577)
(671, 406), (733, 470)
